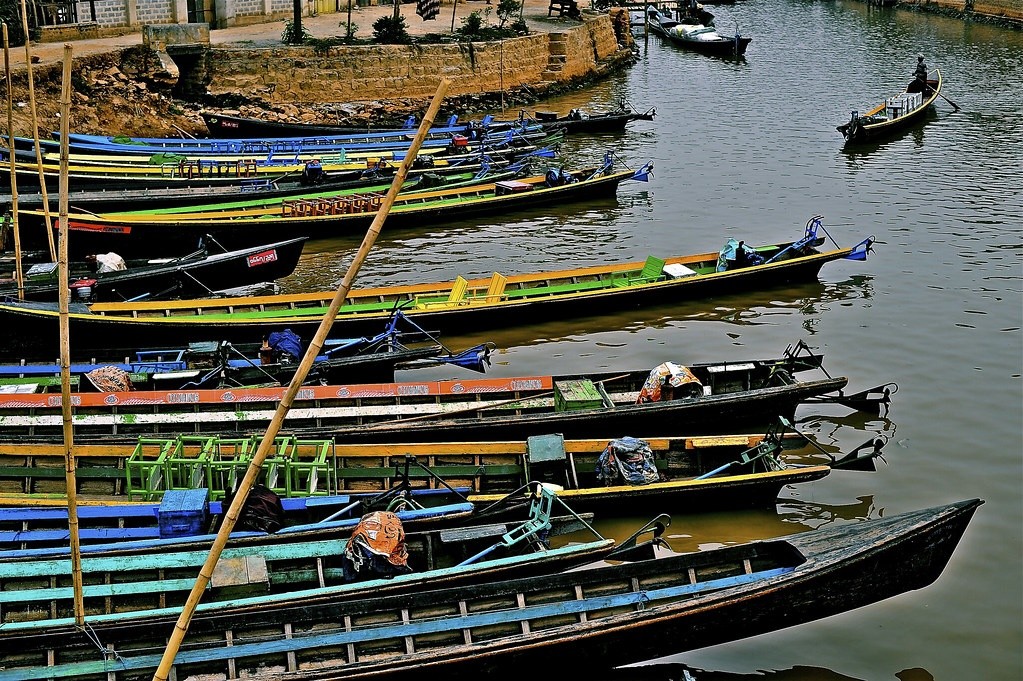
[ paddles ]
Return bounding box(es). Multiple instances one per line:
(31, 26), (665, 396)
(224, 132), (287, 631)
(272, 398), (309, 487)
(905, 69), (961, 111)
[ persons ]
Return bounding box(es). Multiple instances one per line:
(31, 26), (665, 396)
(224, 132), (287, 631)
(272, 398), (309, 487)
(912, 53), (928, 92)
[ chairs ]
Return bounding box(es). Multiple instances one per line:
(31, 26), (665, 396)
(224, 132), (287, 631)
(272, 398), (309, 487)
(402, 116), (416, 129)
(282, 150), (300, 166)
(320, 146), (351, 165)
(481, 114), (495, 125)
(412, 275), (468, 310)
(257, 150), (278, 166)
(460, 271), (509, 306)
(445, 115), (458, 127)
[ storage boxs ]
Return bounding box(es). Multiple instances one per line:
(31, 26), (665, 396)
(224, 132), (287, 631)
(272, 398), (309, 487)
(24, 262), (58, 281)
(158, 488), (212, 538)
(554, 379), (603, 413)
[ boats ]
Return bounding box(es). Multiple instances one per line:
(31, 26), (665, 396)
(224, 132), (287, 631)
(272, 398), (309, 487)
(0, 317), (898, 619)
(835, 68), (942, 143)
(0, 236), (311, 302)
(646, 5), (753, 55)
(0, 497), (984, 681)
(200, 112), (656, 140)
(0, 113), (636, 236)
(0, 212), (875, 346)
